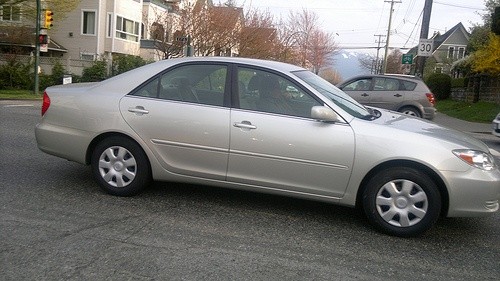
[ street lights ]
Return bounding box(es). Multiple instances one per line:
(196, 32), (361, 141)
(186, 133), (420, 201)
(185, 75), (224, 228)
(283, 30), (309, 64)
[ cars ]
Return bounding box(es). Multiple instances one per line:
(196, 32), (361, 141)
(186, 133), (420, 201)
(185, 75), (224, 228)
(34, 54), (500, 240)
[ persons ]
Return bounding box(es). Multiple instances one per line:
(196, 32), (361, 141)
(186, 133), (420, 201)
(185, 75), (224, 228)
(355, 81), (366, 91)
(256, 76), (296, 115)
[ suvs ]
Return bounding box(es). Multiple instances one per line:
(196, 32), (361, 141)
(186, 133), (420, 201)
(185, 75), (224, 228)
(334, 72), (439, 120)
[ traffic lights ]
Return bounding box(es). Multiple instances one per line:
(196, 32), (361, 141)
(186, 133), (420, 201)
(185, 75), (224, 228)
(45, 10), (54, 30)
(38, 34), (48, 43)
